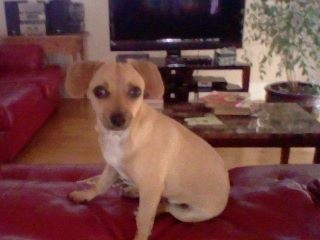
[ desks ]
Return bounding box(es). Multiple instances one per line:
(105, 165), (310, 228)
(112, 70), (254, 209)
(1, 33), (90, 100)
(162, 102), (319, 164)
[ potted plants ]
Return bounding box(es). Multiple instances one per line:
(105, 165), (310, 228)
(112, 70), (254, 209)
(245, 0), (319, 103)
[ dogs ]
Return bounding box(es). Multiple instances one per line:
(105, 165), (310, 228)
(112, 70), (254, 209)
(64, 54), (231, 240)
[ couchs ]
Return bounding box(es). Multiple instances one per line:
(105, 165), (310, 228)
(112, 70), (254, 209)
(0, 44), (64, 162)
(0, 165), (319, 240)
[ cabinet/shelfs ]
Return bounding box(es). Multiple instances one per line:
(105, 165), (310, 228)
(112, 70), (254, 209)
(116, 54), (252, 101)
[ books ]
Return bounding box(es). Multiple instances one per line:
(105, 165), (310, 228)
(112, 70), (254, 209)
(215, 100), (263, 115)
(198, 93), (249, 108)
(185, 117), (225, 130)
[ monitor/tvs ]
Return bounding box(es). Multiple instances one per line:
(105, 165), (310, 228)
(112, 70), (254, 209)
(108, 0), (245, 64)
